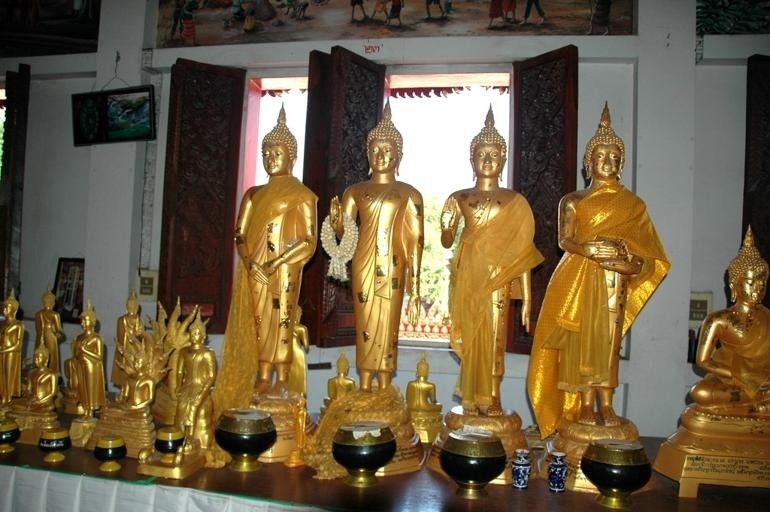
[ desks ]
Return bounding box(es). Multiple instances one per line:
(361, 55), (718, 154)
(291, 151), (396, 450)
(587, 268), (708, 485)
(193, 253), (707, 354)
(0, 431), (768, 508)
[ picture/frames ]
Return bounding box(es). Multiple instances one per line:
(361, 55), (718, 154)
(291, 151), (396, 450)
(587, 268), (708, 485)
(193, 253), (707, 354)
(68, 84), (157, 148)
(50, 257), (86, 323)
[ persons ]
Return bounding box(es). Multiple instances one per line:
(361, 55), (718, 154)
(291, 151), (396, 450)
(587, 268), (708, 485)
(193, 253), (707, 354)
(526, 99), (670, 426)
(441, 105), (543, 419)
(406, 358), (441, 411)
(687, 224), (770, 411)
(0, 292), (218, 449)
(323, 352), (355, 409)
(236, 102), (313, 397)
(320, 102), (425, 442)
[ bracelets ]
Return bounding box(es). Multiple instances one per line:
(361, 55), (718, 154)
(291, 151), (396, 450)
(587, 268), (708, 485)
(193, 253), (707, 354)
(320, 212), (358, 281)
